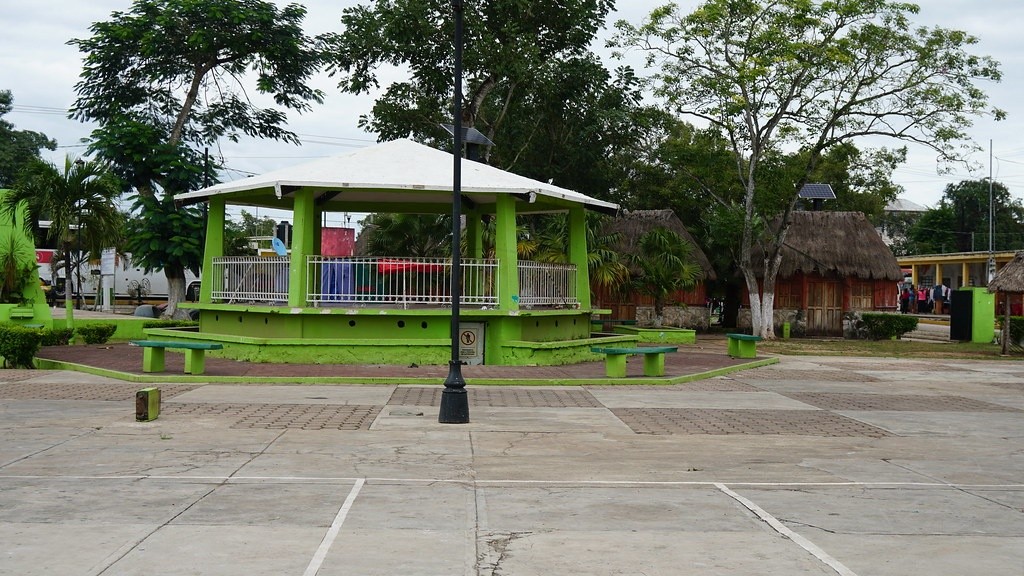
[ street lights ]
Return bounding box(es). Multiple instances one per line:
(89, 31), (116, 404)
(436, 0), (473, 423)
(73, 157), (88, 311)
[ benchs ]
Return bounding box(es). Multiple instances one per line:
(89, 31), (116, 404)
(591, 346), (679, 378)
(590, 319), (639, 332)
(727, 333), (763, 360)
(128, 339), (224, 375)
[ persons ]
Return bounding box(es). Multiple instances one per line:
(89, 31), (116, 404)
(915, 286), (928, 314)
(899, 282), (917, 315)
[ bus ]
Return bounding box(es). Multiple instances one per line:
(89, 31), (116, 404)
(31, 219), (201, 300)
